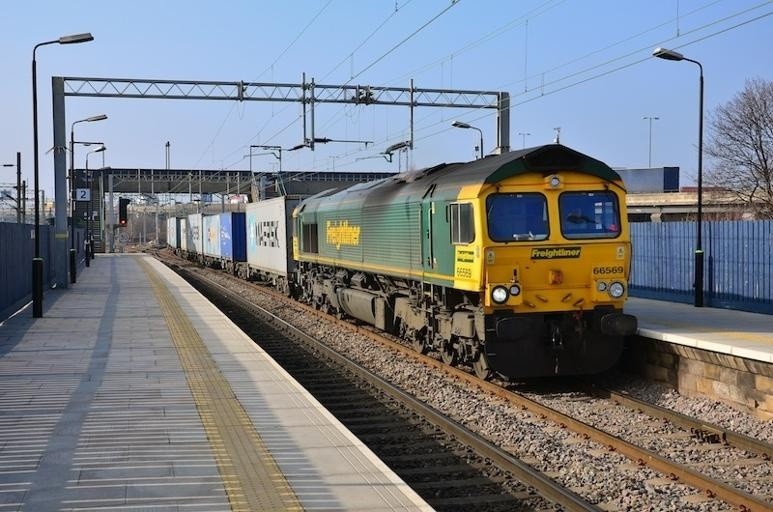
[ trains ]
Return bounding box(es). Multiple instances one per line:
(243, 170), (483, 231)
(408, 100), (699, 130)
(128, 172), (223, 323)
(167, 144), (637, 388)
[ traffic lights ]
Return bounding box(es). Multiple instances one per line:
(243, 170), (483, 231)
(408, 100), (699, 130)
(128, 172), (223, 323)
(119, 197), (130, 227)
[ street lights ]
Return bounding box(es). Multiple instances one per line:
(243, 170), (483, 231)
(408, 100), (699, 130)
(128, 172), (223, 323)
(32, 33), (107, 320)
(517, 132), (531, 148)
(451, 120), (483, 159)
(653, 48), (704, 307)
(641, 114), (661, 168)
